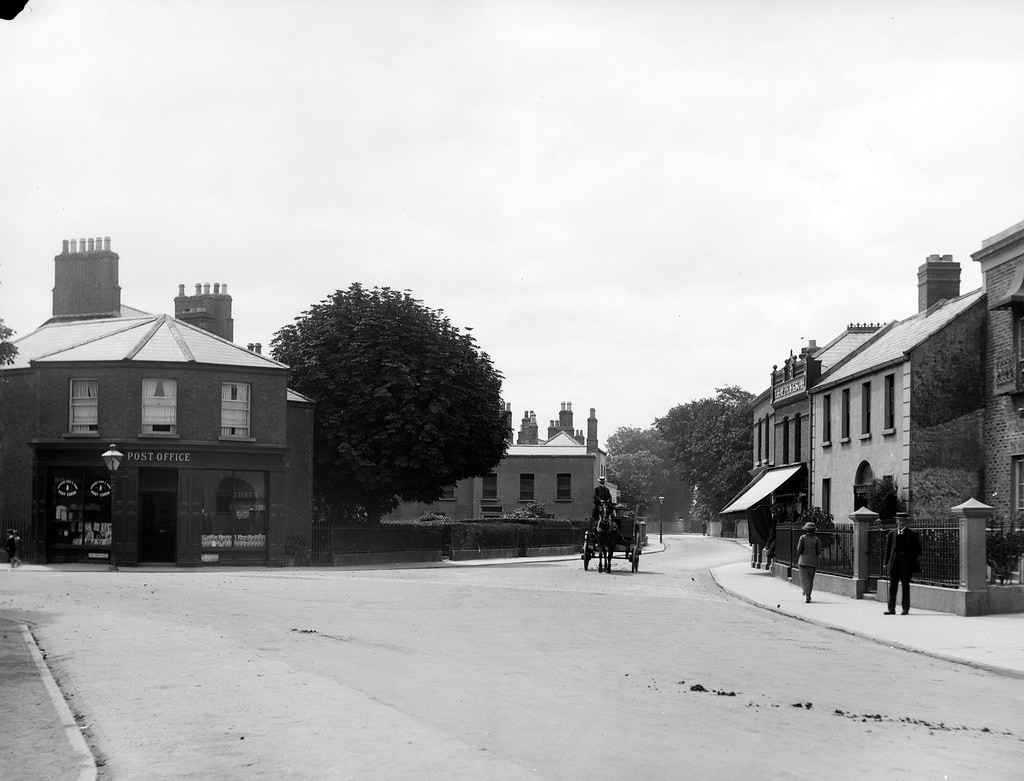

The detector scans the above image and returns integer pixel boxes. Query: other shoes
[901,610,909,615]
[883,610,895,614]
[805,594,811,603]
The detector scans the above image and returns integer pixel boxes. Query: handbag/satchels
[799,542,804,553]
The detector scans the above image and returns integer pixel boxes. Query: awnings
[718,465,806,514]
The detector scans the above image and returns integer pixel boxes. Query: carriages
[582,495,642,575]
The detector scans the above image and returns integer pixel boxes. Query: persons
[702,521,707,535]
[592,477,614,529]
[884,513,919,616]
[5,529,21,568]
[797,522,822,603]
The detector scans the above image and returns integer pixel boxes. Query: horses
[594,495,619,572]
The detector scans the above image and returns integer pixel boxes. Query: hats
[802,522,818,532]
[598,477,607,482]
[894,512,911,519]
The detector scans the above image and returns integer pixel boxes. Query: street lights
[658,490,664,544]
[100,443,124,572]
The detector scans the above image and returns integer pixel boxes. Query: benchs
[589,516,634,540]
